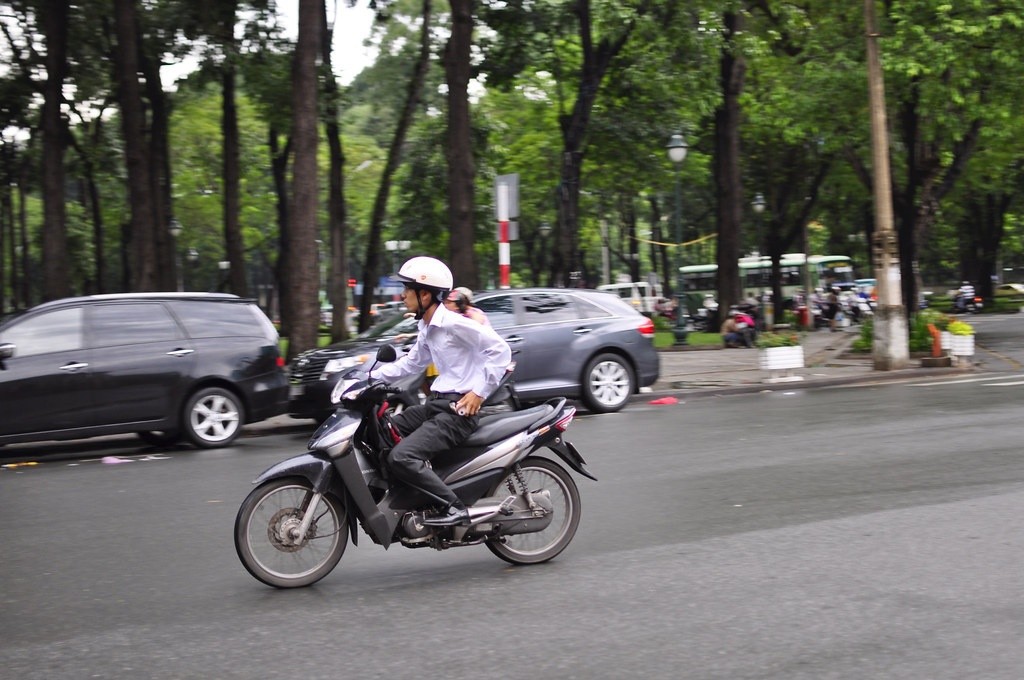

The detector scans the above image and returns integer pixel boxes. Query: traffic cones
[931,330,943,358]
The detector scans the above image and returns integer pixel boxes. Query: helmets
[454,287,473,301]
[445,291,468,304]
[389,256,453,292]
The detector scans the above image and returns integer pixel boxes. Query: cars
[288,287,662,428]
[0,292,290,451]
[516,288,655,346]
[595,282,662,318]
[998,283,1024,291]
[854,279,877,290]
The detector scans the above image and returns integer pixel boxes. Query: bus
[678,253,855,322]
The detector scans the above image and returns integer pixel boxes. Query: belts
[431,391,466,402]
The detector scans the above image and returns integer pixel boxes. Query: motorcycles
[384,334,525,419]
[233,344,598,590]
[952,295,983,314]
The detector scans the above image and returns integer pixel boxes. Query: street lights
[666,129,695,346]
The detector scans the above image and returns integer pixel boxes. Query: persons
[364,256,511,526]
[654,298,685,329]
[847,286,878,322]
[954,281,975,309]
[721,310,758,348]
[817,271,843,332]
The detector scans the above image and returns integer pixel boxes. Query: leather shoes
[420,505,471,527]
